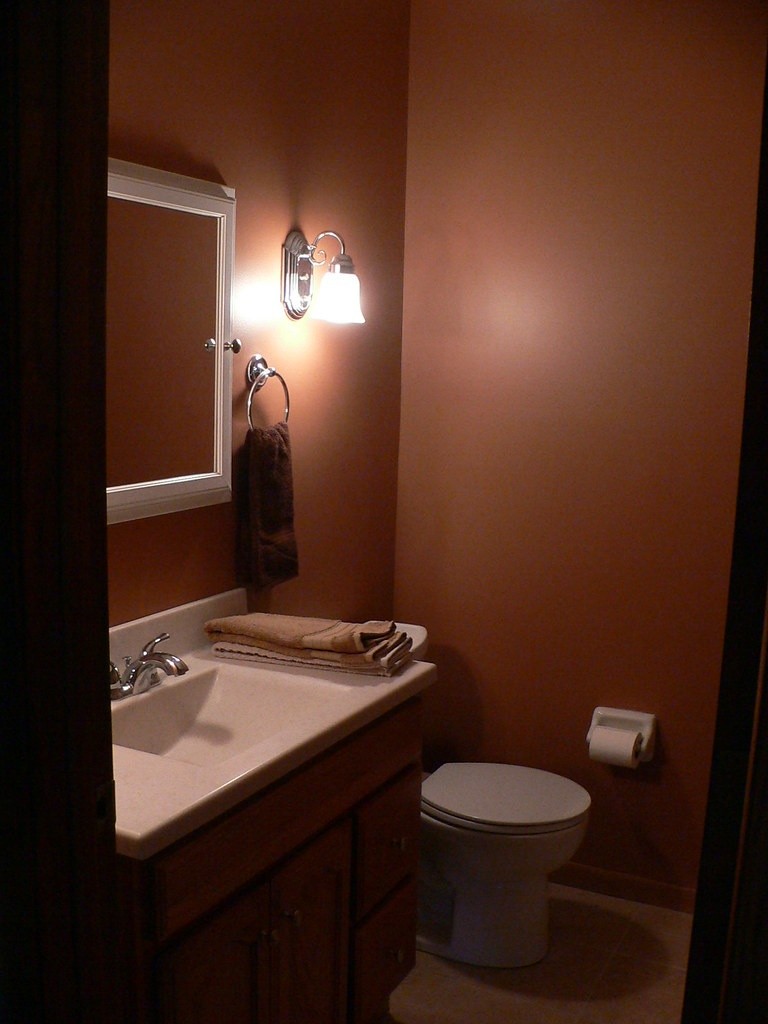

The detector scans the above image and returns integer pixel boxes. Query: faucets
[121,651,189,694]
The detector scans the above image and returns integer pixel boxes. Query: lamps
[280,230,365,324]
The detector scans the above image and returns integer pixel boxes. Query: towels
[202,611,415,679]
[233,422,300,593]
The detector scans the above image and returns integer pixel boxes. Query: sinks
[112,664,352,769]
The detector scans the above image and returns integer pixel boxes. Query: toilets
[360,619,591,969]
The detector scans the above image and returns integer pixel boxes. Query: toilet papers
[589,725,644,769]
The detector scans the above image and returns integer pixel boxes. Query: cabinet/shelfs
[120,696,427,1024]
